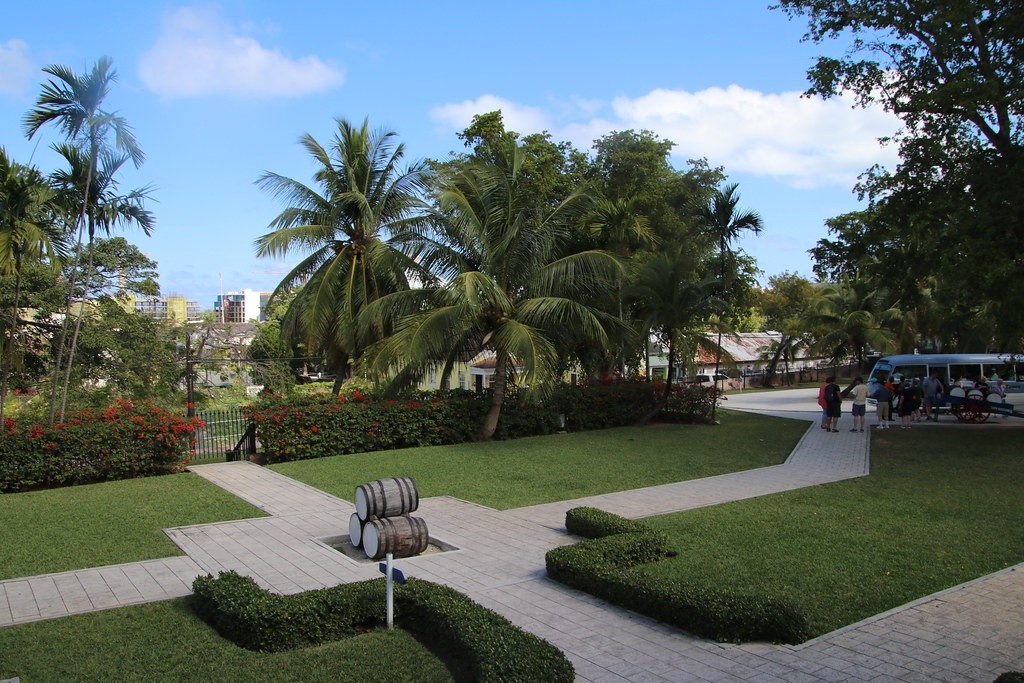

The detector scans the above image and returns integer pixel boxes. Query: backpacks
[824,386,835,403]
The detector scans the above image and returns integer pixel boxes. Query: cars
[697,374,730,384]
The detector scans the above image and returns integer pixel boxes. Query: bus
[865,353,1024,412]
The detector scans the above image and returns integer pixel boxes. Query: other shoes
[820,425,826,429]
[832,429,840,432]
[826,428,831,432]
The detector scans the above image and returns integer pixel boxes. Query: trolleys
[942,384,1024,424]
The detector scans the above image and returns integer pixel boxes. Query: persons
[819,378,830,429]
[849,377,870,432]
[872,380,891,429]
[887,376,897,420]
[825,375,842,432]
[897,371,1005,430]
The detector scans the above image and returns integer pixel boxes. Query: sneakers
[883,426,889,429]
[900,425,906,428]
[859,428,864,432]
[849,428,858,432]
[908,426,911,428]
[876,426,884,429]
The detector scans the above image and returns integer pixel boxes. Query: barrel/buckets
[355,477,419,522]
[362,516,428,559]
[349,513,370,548]
